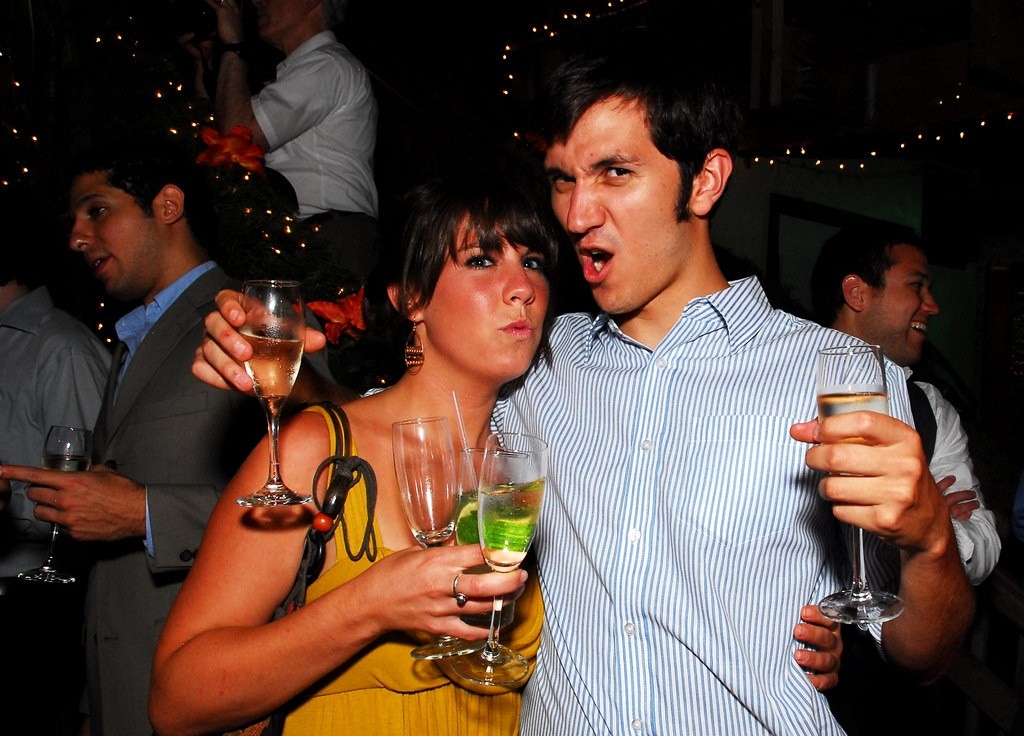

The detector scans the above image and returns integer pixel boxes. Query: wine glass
[235,278,313,511]
[818,344,905,627]
[16,424,93,587]
[451,433,549,687]
[392,416,484,665]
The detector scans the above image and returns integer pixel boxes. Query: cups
[455,448,528,629]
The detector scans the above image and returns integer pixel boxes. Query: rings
[453,575,471,606]
[218,0,225,3]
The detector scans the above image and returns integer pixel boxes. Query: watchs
[222,42,250,60]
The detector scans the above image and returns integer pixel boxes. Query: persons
[149,167,845,736]
[189,37,973,736]
[792,223,1024,736]
[0,1,378,736]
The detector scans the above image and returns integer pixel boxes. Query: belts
[299,209,364,226]
